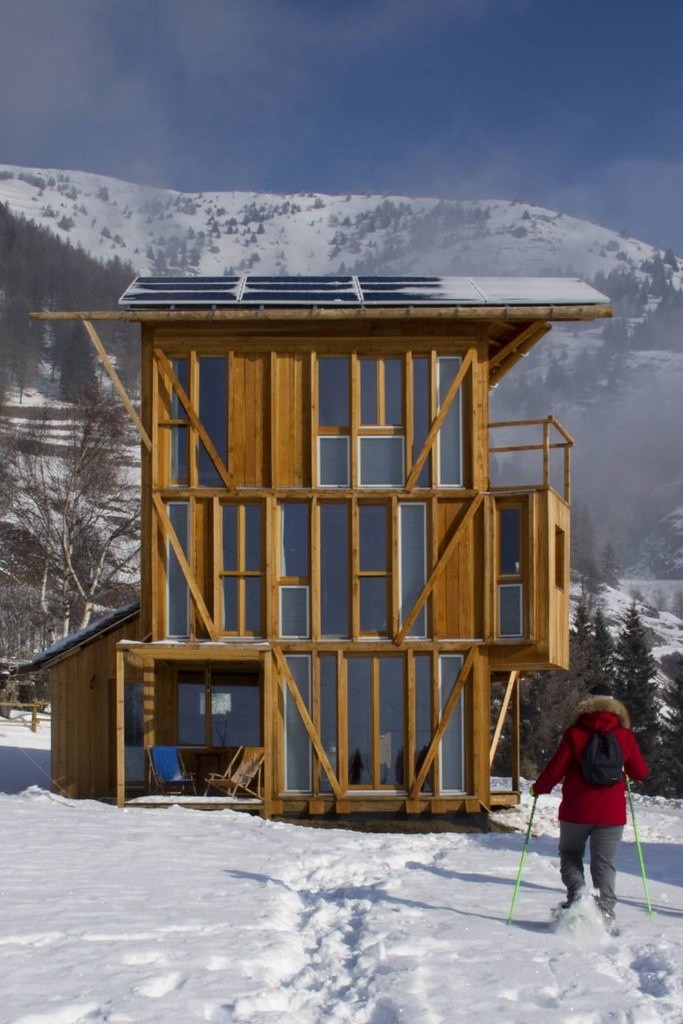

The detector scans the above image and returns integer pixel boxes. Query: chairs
[144,744,197,796]
[203,745,266,801]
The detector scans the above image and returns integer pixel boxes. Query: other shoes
[605,925,619,937]
[561,893,584,909]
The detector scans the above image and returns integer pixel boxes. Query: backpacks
[571,722,624,786]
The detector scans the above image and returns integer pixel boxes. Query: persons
[529,683,649,926]
